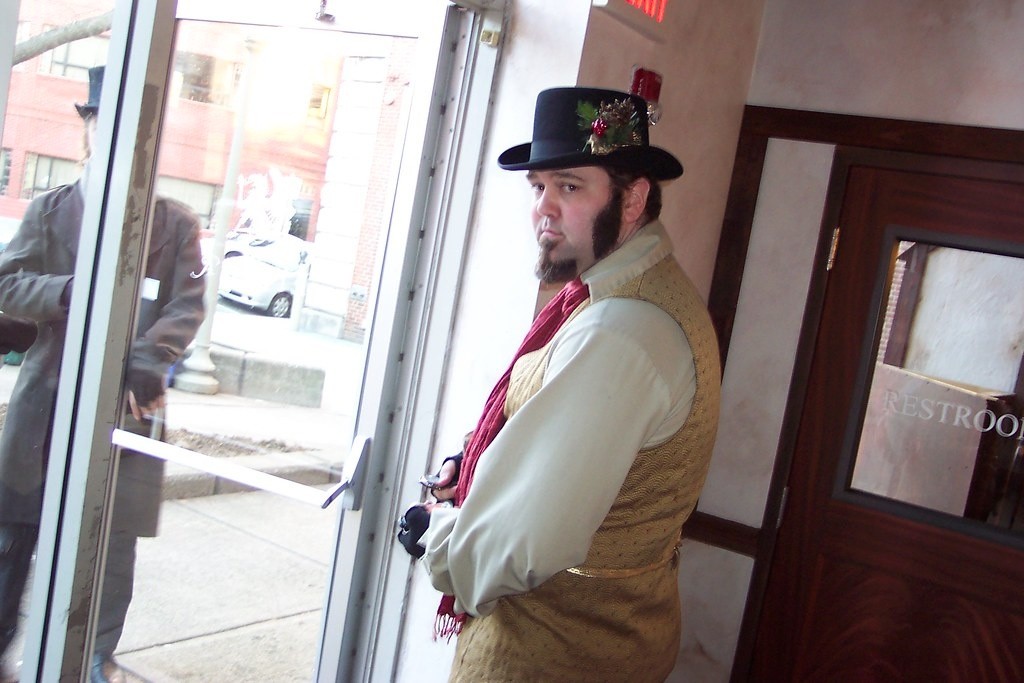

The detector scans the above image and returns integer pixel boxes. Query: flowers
[573,92,644,157]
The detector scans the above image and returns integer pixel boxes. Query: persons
[398,86,721,683]
[0,64,207,683]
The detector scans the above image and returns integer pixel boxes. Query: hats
[74,66,105,118]
[497,86,684,181]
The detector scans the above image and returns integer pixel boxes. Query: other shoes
[91,653,126,683]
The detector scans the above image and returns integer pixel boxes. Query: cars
[191,208,313,320]
